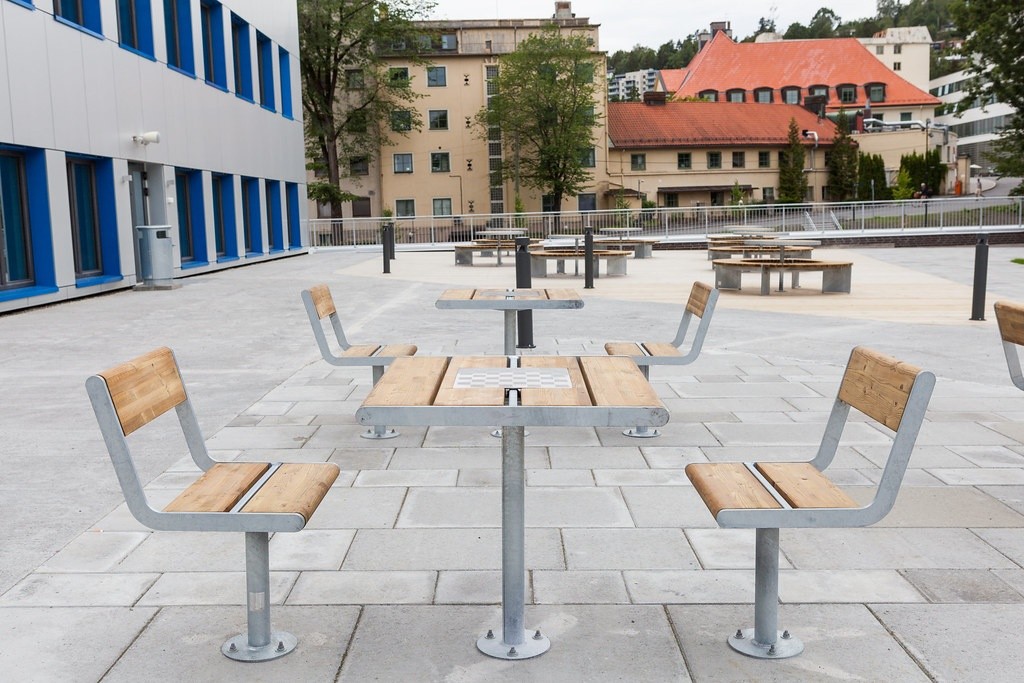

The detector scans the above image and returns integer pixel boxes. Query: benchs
[458,235,657,279]
[708,236,853,297]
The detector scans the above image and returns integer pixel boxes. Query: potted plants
[672,212,684,223]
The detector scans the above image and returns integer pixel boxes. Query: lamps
[468,200,475,212]
[465,115,471,127]
[466,158,473,171]
[132,131,160,145]
[464,73,469,85]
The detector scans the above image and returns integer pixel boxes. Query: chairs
[606,280,718,437]
[684,345,937,660]
[303,285,419,438]
[995,304,1024,392]
[86,345,340,659]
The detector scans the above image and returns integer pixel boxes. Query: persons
[917,183,928,207]
[976,179,985,201]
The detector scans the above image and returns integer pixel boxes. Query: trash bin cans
[136,225,173,285]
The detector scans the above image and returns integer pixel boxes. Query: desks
[357,354,671,660]
[485,228,528,256]
[435,287,583,356]
[551,234,609,275]
[724,225,822,290]
[601,227,642,251]
[475,231,526,263]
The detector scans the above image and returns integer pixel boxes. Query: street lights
[926,118,931,158]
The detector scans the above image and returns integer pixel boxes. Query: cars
[979,168,1003,176]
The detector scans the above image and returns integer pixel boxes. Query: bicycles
[912,193,933,207]
